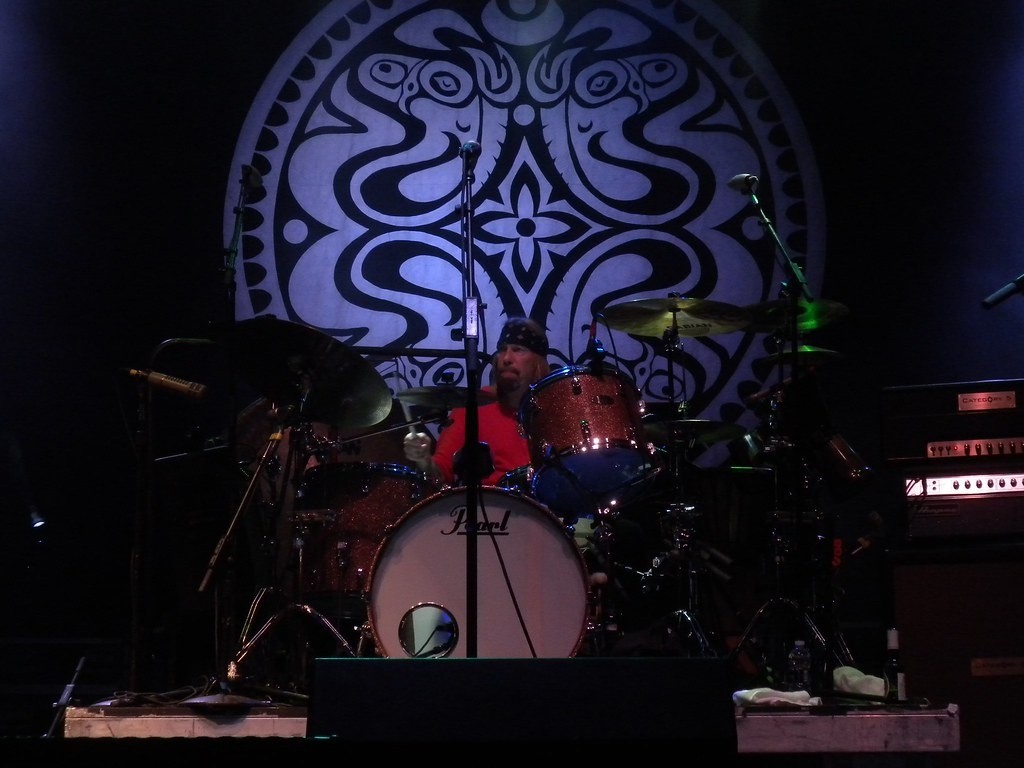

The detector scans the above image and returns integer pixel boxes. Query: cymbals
[395,384,497,409]
[740,293,854,337]
[216,316,395,429]
[769,344,840,362]
[595,296,756,340]
[640,417,747,449]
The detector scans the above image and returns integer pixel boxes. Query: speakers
[879,543,1024,766]
[305,657,739,768]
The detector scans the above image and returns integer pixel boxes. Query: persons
[404,317,553,486]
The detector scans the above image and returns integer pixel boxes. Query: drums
[513,365,656,506]
[365,483,592,659]
[294,460,452,622]
[495,462,540,500]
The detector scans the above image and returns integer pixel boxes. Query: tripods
[111,365,361,708]
[658,430,860,672]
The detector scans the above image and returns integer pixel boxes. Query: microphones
[265,405,296,420]
[585,314,597,360]
[749,368,816,402]
[459,141,482,159]
[124,366,208,402]
[241,164,264,190]
[725,174,759,192]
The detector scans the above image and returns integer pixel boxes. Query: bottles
[881,623,908,707]
[789,641,811,694]
